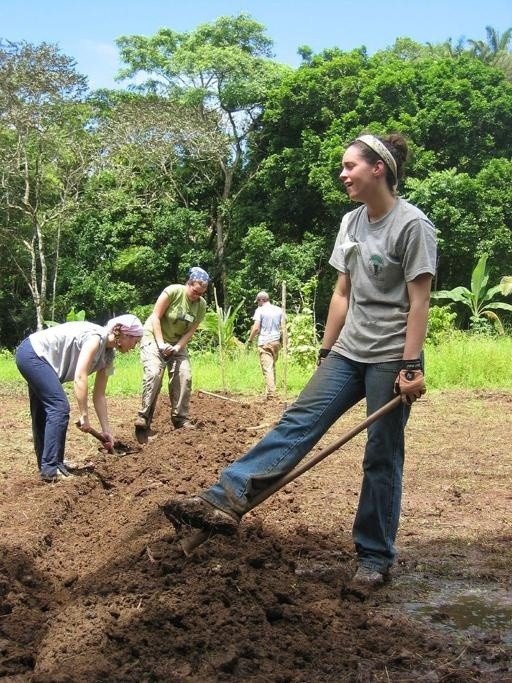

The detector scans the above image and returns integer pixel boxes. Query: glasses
[190,285,205,296]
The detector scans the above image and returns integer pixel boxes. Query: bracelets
[247,340,252,343]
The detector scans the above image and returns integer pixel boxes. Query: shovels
[135,348,174,444]
[75,420,140,456]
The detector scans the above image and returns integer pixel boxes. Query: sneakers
[135,417,148,429]
[41,459,80,483]
[166,496,238,537]
[177,421,196,430]
[352,566,385,589]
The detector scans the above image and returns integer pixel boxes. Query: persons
[245,291,283,400]
[15,314,143,481]
[162,134,437,583]
[134,266,209,429]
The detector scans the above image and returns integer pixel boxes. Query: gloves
[245,340,252,351]
[393,359,425,406]
